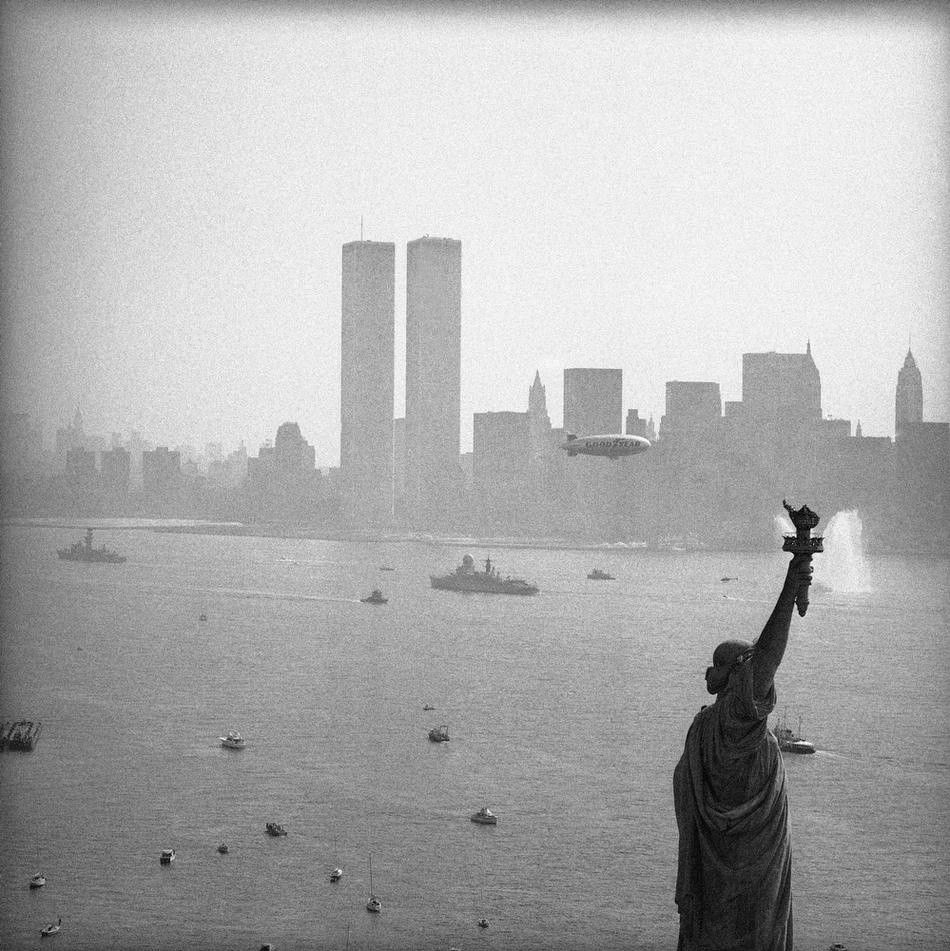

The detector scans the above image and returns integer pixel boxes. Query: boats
[219,729,247,749]
[771,707,817,753]
[57,527,128,563]
[423,702,452,741]
[40,918,61,935]
[160,849,177,864]
[362,590,388,605]
[430,552,539,596]
[218,842,229,853]
[472,807,497,825]
[587,568,616,580]
[380,565,395,570]
[266,822,289,836]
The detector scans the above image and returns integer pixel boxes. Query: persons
[673,552,815,951]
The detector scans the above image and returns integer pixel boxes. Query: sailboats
[478,889,490,927]
[330,834,342,881]
[29,829,46,887]
[364,851,381,911]
